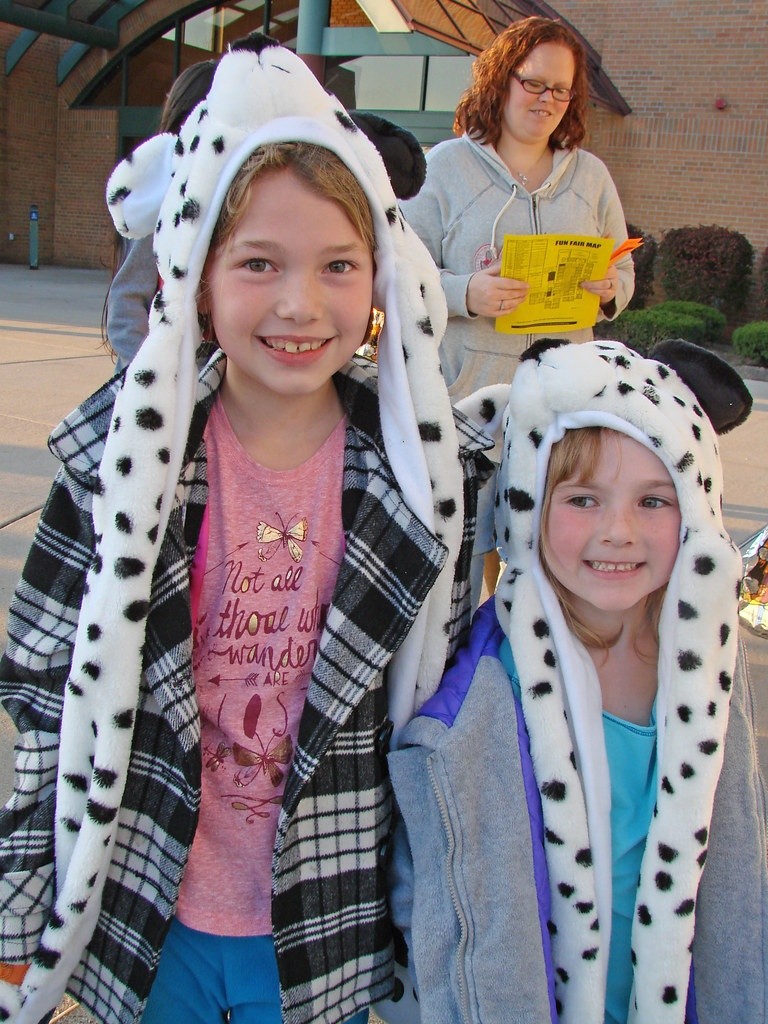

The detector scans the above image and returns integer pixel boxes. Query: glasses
[511,68,580,102]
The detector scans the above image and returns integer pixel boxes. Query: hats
[445,335,754,1024]
[4,33,463,1024]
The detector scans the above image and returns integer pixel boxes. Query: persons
[398,16,635,602]
[385,337,767,1024]
[0,34,464,1023]
